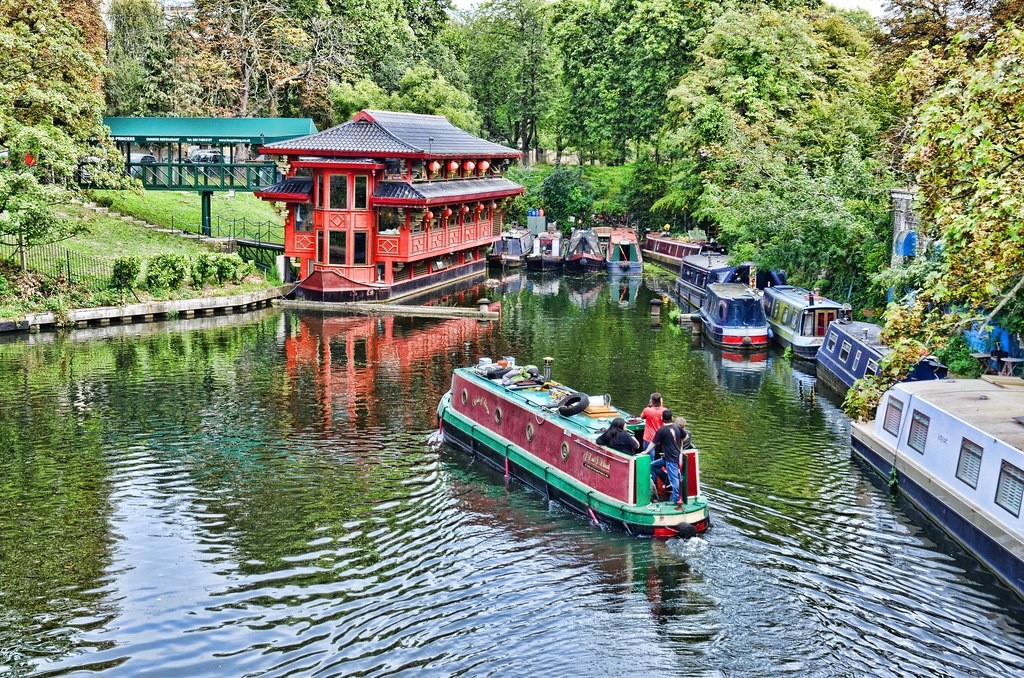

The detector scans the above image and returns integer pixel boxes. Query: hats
[662,410,671,420]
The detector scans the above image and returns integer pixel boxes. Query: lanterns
[464,161,475,173]
[442,208,452,220]
[476,204,484,212]
[423,210,433,221]
[447,161,459,172]
[460,205,469,215]
[478,161,490,172]
[429,162,439,173]
[490,203,496,210]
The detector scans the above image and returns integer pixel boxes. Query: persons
[675,417,692,451]
[642,410,689,504]
[641,392,671,491]
[596,418,640,455]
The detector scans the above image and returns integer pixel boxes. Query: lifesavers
[557,393,590,417]
[487,367,513,379]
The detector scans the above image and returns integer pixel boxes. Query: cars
[66,147,230,186]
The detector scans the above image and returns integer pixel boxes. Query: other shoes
[665,502,678,506]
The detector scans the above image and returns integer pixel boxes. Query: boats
[640,223,729,268]
[674,250,787,311]
[436,354,711,538]
[699,282,771,351]
[815,305,950,416]
[845,371,1024,603]
[760,283,853,359]
[488,222,645,274]
